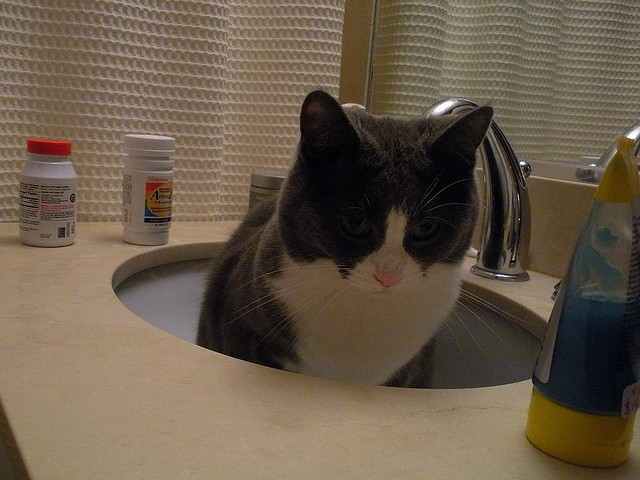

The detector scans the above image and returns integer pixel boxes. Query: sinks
[111,242,548,389]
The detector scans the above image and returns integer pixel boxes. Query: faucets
[419,98,532,282]
[551,137,640,303]
[577,122,640,182]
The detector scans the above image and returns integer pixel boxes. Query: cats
[195,90,494,387]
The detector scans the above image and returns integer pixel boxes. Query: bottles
[120,133,176,245]
[248,171,286,209]
[18,138,79,247]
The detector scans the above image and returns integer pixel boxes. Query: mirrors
[365,0,640,185]
